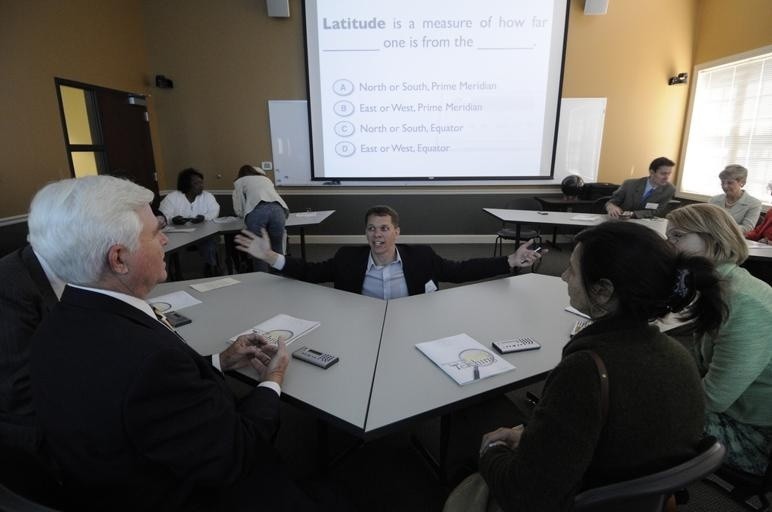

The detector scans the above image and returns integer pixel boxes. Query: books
[227,314,321,354]
[145,289,203,314]
[414,333,516,386]
[160,226,195,233]
[569,319,592,336]
[744,238,771,250]
[566,306,592,321]
[211,215,237,224]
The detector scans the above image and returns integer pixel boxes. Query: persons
[604,158,676,223]
[660,203,771,476]
[234,205,549,300]
[439,220,731,511]
[158,168,221,275]
[231,165,290,275]
[2,178,77,501]
[27,174,341,512]
[742,182,771,248]
[112,169,166,230]
[705,164,764,235]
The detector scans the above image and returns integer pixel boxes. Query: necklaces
[724,200,737,207]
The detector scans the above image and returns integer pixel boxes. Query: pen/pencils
[520,247,542,264]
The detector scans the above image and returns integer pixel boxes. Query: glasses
[668,231,696,245]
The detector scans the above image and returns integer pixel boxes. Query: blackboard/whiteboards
[267,97,607,187]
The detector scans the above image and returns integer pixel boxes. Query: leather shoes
[676,488,689,505]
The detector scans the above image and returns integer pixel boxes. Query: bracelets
[480,441,510,457]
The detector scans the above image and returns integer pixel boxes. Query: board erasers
[323,181,340,186]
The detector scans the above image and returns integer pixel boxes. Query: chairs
[567,433,724,510]
[494,197,541,257]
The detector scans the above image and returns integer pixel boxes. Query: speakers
[267,0,290,17]
[584,0,608,15]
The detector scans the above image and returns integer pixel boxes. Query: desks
[218,207,336,269]
[534,196,681,253]
[483,206,772,280]
[141,269,387,508]
[159,220,219,279]
[365,270,686,510]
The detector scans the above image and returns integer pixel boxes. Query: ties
[150,304,185,341]
[640,188,655,203]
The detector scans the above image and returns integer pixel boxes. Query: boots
[204,262,223,277]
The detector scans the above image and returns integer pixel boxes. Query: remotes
[521,247,541,263]
